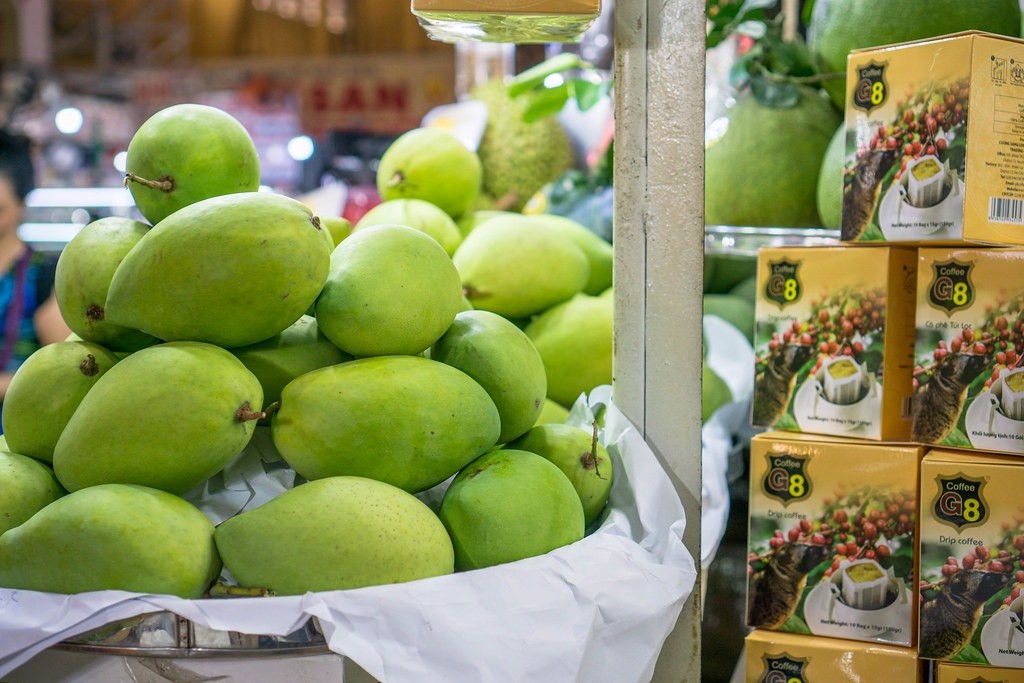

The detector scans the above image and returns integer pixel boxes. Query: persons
[0,128,73,436]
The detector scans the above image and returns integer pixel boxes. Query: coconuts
[707,0,1024,232]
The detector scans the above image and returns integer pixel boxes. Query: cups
[1004,593,1024,654]
[824,568,902,637]
[894,169,955,235]
[985,376,1024,444]
[809,367,873,431]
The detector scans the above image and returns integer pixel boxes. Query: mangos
[1,103,759,602]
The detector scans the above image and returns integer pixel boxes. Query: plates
[878,178,964,239]
[965,391,1024,453]
[981,607,1024,668]
[804,580,912,646]
[793,377,882,441]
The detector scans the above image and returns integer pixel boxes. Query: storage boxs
[749,245,913,443]
[745,438,924,648]
[937,662,1024,683]
[744,629,919,683]
[917,451,1024,668]
[912,247,1024,456]
[840,30,1023,249]
